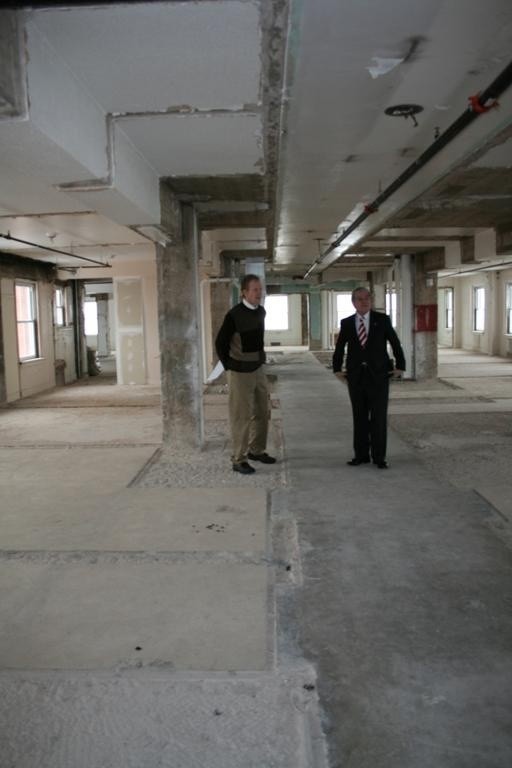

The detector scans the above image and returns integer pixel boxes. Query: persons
[333,288,406,469]
[214,275,276,474]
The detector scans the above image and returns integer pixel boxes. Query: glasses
[355,297,369,301]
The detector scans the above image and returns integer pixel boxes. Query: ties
[357,317,368,349]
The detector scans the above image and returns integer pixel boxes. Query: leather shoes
[373,458,389,469]
[346,456,370,466]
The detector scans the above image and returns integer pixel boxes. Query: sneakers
[233,462,256,474]
[246,452,277,464]
